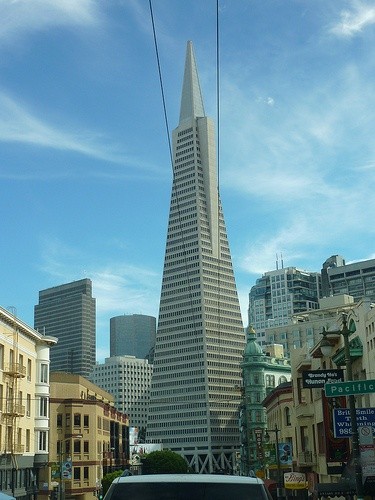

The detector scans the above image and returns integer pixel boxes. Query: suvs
[101,474,269,500]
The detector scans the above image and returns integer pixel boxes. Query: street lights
[264,425,282,500]
[59,435,83,500]
[318,314,365,500]
